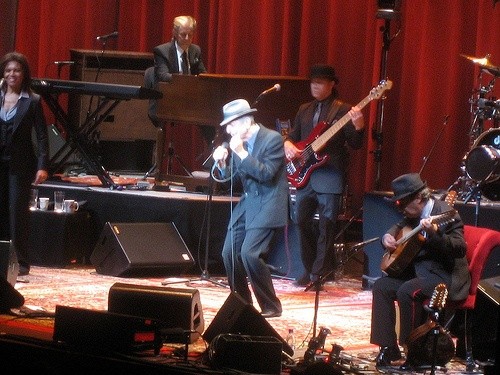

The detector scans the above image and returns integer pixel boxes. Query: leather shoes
[401,359,411,370]
[375,344,401,365]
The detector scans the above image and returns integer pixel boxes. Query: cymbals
[457,52,500,76]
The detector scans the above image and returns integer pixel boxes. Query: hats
[391,173,425,203]
[309,65,339,81]
[220,98,258,127]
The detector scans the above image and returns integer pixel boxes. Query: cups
[64,200,79,213]
[54,191,65,212]
[39,198,49,210]
[29,188,40,210]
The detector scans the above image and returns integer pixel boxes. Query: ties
[313,103,323,128]
[180,51,188,74]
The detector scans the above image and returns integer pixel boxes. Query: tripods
[161,94,268,289]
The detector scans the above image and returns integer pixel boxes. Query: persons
[147,16,216,191]
[284,64,365,286]
[213,99,288,317]
[371,173,474,371]
[0,52,50,277]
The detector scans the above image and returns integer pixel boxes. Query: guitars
[405,282,456,372]
[285,77,393,191]
[380,209,459,277]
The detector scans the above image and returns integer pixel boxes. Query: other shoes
[260,310,281,317]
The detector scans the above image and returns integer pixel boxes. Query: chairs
[412,224,500,359]
[141,66,194,181]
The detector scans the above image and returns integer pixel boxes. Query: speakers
[0,240,25,312]
[89,223,196,278]
[67,49,159,142]
[470,276,500,362]
[54,282,294,375]
[48,124,82,164]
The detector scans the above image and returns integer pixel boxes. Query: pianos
[154,72,316,198]
[29,77,165,191]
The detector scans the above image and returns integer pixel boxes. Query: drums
[463,145,500,184]
[469,127,500,202]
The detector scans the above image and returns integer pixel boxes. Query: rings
[43,178,45,181]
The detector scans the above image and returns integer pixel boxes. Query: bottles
[285,328,296,356]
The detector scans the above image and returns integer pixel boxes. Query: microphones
[53,61,74,65]
[0,77,5,88]
[96,32,119,40]
[261,83,281,95]
[356,237,380,248]
[211,142,229,172]
[428,283,449,334]
